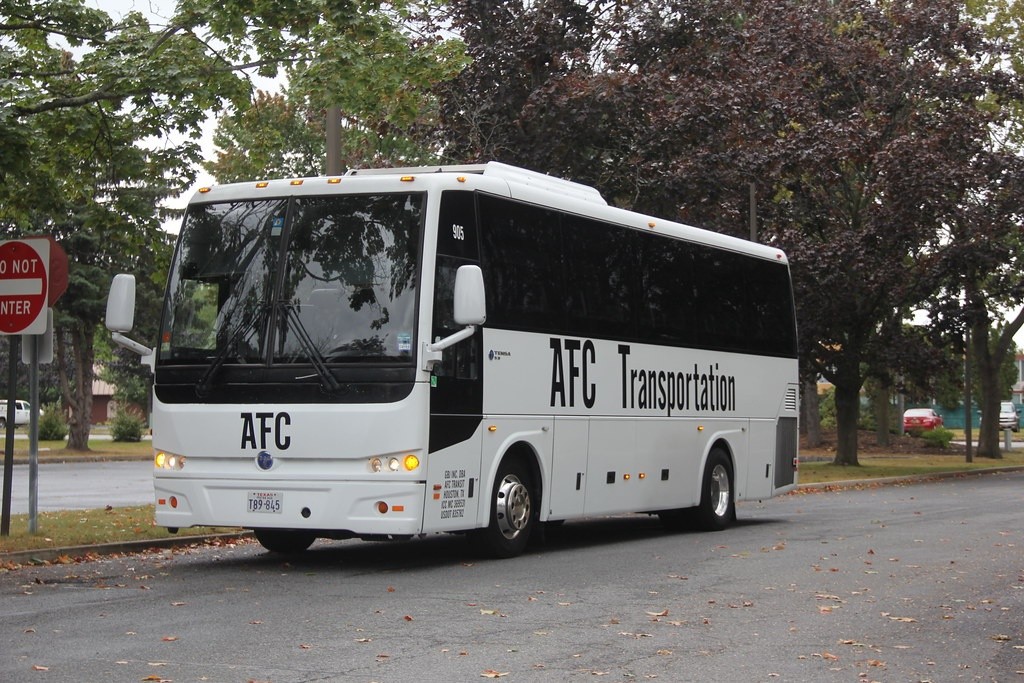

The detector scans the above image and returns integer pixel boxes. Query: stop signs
[0,237,53,337]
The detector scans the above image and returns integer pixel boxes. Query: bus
[104,159,801,559]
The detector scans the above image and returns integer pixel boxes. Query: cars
[977,401,1022,433]
[902,409,945,432]
